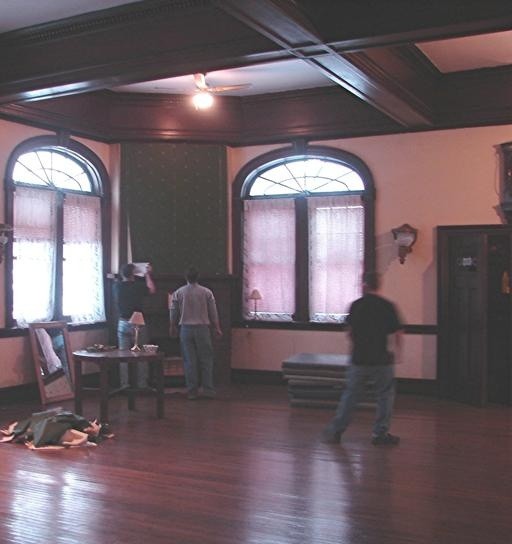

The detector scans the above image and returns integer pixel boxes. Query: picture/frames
[27,321,79,405]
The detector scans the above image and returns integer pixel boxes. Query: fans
[154,77,258,97]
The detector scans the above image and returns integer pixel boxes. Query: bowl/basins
[142,345,158,352]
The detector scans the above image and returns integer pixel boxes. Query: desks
[73,347,172,425]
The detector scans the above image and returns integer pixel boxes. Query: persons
[319,268,404,447]
[112,262,159,389]
[167,265,226,401]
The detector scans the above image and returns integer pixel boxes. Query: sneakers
[186,387,217,400]
[371,431,401,446]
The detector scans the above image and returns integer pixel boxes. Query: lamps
[129,309,146,350]
[251,287,261,322]
[191,92,214,111]
[391,219,418,263]
